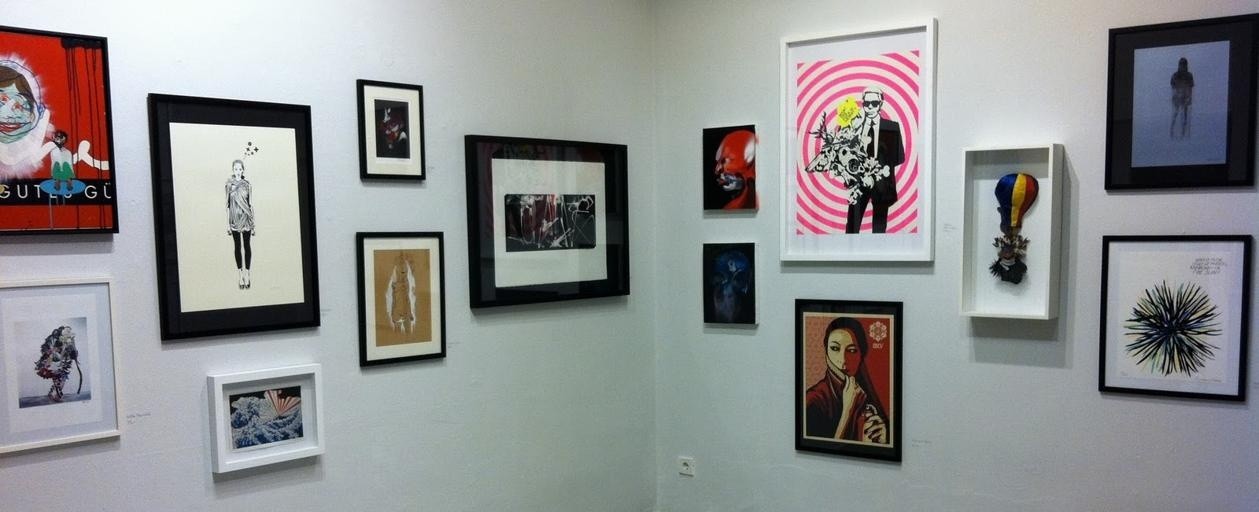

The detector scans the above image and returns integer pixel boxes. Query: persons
[839,85,906,234]
[1165,55,1194,146]
[805,317,889,444]
[224,159,256,290]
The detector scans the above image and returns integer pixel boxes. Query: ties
[867,120,874,157]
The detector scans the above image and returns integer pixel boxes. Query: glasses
[862,101,880,107]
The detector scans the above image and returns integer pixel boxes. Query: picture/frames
[958,143,1064,321]
[1105,13,1259,190]
[206,363,325,474]
[0,25,120,234]
[702,125,756,211]
[779,17,937,263]
[0,277,121,455]
[463,134,629,308]
[147,93,321,342]
[1098,234,1252,401]
[356,232,446,366]
[794,299,903,462]
[702,242,756,326]
[356,79,426,180]
